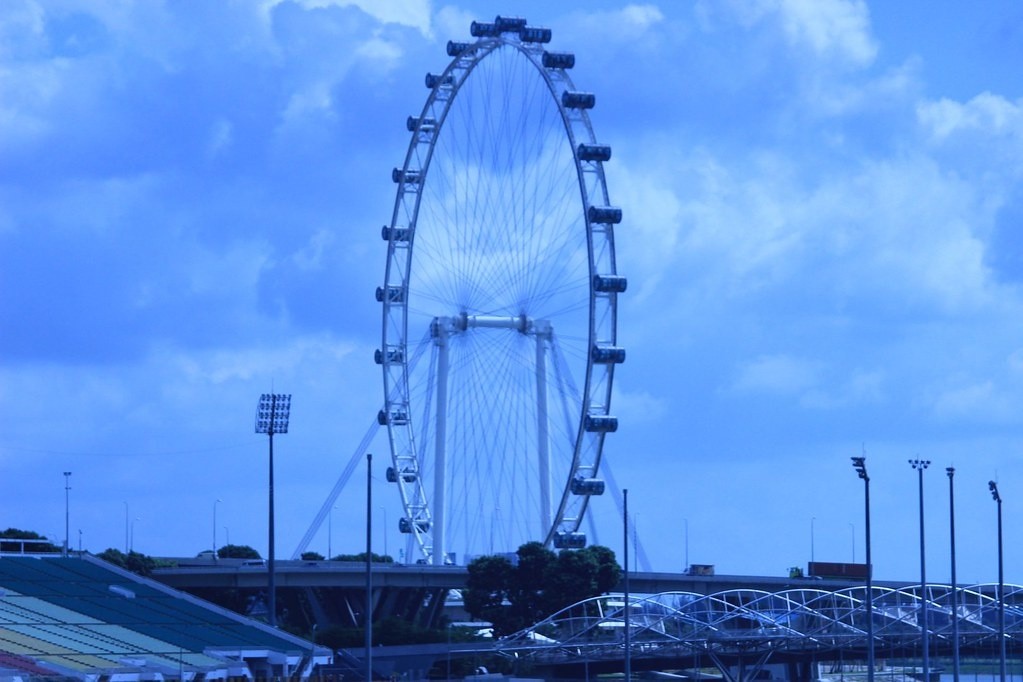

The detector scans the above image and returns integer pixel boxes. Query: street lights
[682,516,691,572]
[631,511,641,573]
[212,498,223,558]
[379,505,387,564]
[64,470,73,556]
[909,459,930,682]
[131,518,141,551]
[222,526,230,558]
[850,456,875,682]
[254,392,293,627]
[945,463,962,682]
[79,529,83,556]
[329,504,340,561]
[809,517,817,579]
[849,524,857,565]
[122,501,128,556]
[988,480,1006,682]
